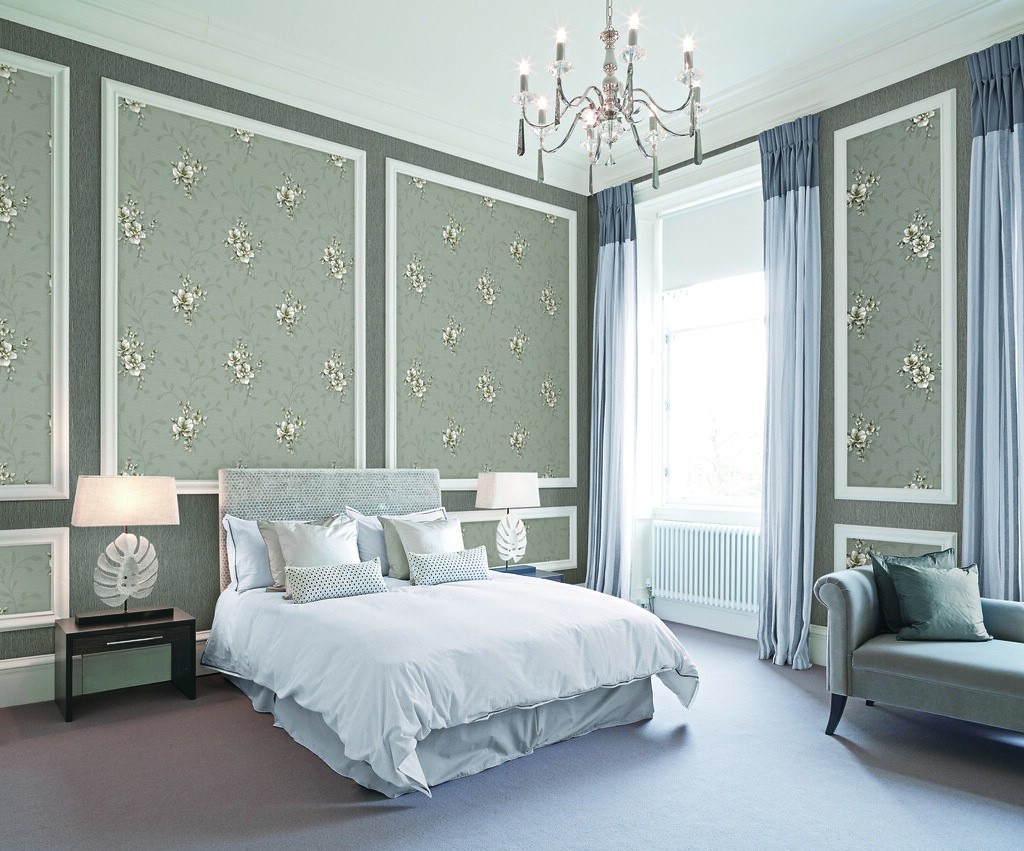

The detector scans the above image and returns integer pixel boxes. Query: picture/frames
[450,506,577,571]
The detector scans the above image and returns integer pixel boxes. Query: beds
[200,468,699,798]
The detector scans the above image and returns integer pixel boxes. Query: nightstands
[533,569,565,583]
[54,607,197,723]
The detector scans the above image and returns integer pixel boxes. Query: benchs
[814,564,1024,737]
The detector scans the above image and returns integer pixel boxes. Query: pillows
[345,505,447,576]
[885,562,993,642]
[257,515,340,592]
[283,556,387,605]
[391,519,465,554]
[377,506,447,579]
[406,544,493,584]
[867,547,956,633]
[274,518,361,601]
[222,514,275,592]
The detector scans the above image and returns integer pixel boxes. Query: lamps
[475,470,541,576]
[516,0,703,197]
[70,476,181,626]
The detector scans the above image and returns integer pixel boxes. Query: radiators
[649,518,760,614]
